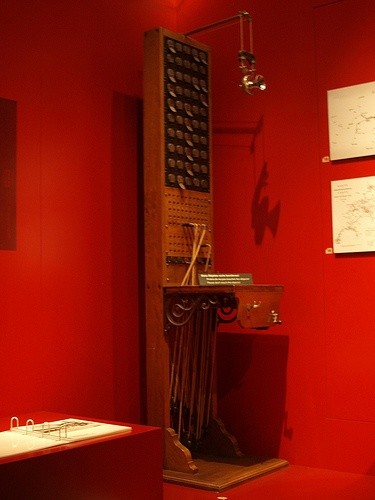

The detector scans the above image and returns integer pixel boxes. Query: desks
[0,414,163,500]
